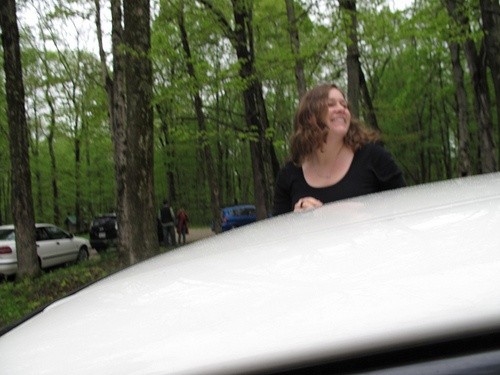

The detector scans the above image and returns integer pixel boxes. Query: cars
[211,205,272,234]
[0,170,500,375]
[0,221,91,281]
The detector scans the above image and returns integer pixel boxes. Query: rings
[300,201,304,208]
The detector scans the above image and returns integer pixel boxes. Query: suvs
[87,212,120,256]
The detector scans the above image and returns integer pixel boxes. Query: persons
[176,202,189,246]
[157,200,178,248]
[273,83,406,218]
